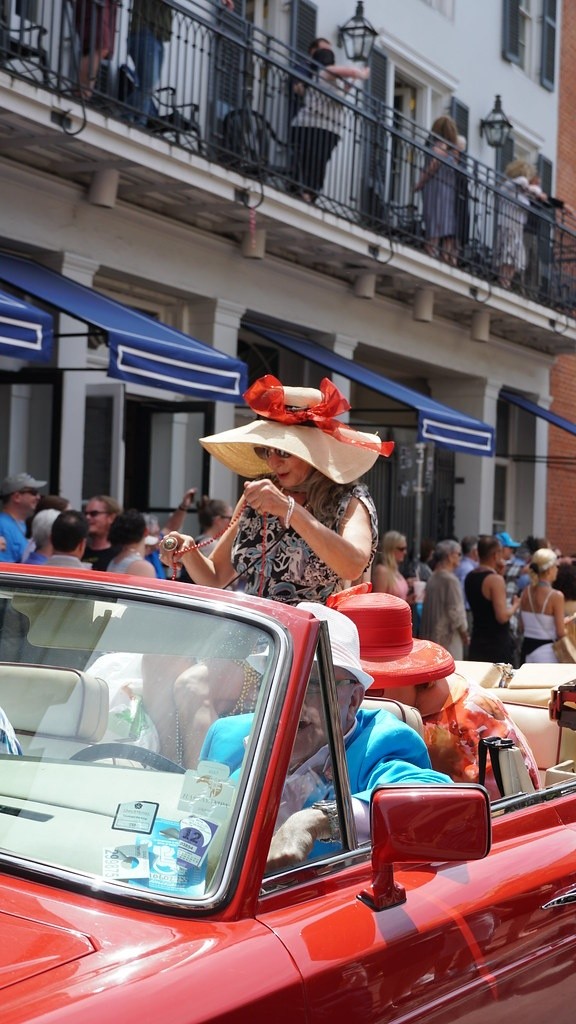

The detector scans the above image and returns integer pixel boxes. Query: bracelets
[285,495,295,529]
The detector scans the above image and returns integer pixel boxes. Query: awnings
[500,388,576,465]
[0,290,56,363]
[240,319,496,459]
[0,251,248,404]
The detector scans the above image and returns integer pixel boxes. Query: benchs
[85,650,575,787]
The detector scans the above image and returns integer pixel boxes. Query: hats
[0,473,47,495]
[326,582,455,690]
[497,533,522,548]
[198,373,394,485]
[530,548,556,569]
[245,600,373,695]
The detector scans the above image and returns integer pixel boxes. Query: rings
[161,537,178,552]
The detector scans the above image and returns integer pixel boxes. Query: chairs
[365,173,425,252]
[118,66,202,154]
[460,238,576,316]
[0,0,50,87]
[224,109,299,193]
[0,660,116,765]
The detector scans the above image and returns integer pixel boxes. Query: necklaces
[172,499,267,600]
[278,486,313,509]
[176,655,259,769]
[122,545,141,557]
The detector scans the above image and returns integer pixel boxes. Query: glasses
[84,510,112,517]
[19,489,39,496]
[394,546,406,552]
[252,444,292,461]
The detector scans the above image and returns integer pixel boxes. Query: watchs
[311,800,340,844]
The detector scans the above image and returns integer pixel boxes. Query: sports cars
[0,562,575,1024]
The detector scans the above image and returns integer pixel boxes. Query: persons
[491,159,576,291]
[142,374,378,771]
[412,116,459,262]
[66,0,173,128]
[197,593,541,892]
[285,37,371,202]
[0,472,575,672]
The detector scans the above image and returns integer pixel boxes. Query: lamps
[336,0,379,63]
[481,94,513,149]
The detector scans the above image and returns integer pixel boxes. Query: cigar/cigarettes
[298,719,308,732]
